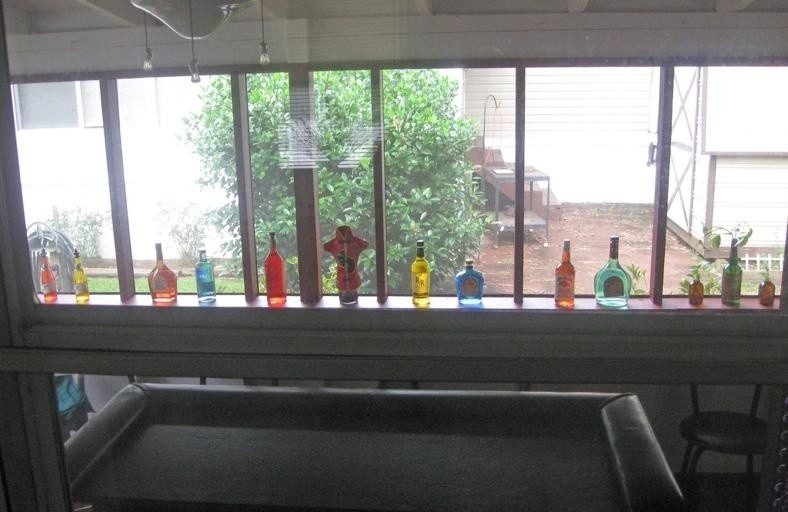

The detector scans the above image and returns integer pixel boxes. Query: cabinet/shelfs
[485,165,551,248]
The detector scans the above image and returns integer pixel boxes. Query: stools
[679,410,768,511]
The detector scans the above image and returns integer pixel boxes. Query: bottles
[263,231,288,308]
[593,235,632,308]
[719,238,743,306]
[411,238,430,305]
[453,259,485,305]
[554,238,575,311]
[194,250,216,304]
[38,247,58,304]
[70,248,91,304]
[688,278,705,306]
[147,244,178,303]
[757,279,776,306]
